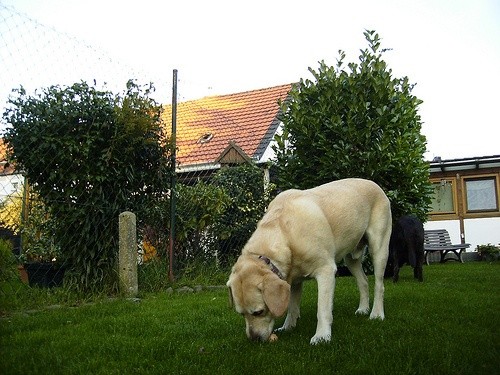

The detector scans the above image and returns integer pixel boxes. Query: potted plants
[474,244,500,261]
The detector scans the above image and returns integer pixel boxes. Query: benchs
[423,228,470,266]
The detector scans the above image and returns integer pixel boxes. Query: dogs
[384,216,424,283]
[226,178,392,346]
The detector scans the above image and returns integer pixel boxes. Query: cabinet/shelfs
[18,263,63,288]
